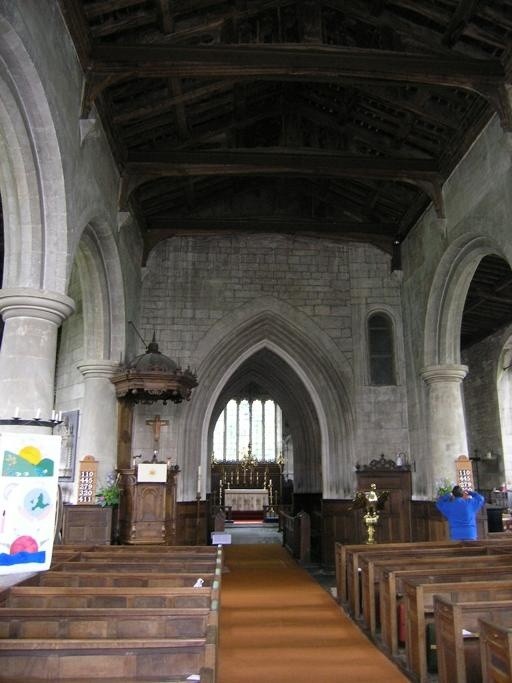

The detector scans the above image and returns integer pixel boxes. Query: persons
[435,485,485,541]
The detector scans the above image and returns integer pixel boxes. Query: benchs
[0,541,224,683]
[335,538,512,683]
